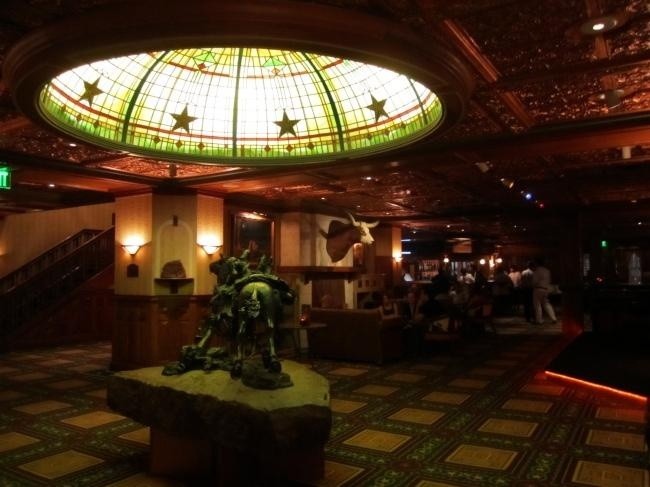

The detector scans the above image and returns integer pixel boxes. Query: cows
[326,212,380,263]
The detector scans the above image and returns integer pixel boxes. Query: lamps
[120,243,141,255]
[199,244,222,254]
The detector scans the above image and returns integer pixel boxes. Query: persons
[381,256,555,325]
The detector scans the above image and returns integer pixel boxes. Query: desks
[278,322,329,367]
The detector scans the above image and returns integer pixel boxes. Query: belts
[534,286,548,290]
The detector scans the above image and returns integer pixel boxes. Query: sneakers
[528,318,558,329]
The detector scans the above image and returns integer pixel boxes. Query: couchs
[304,305,407,361]
